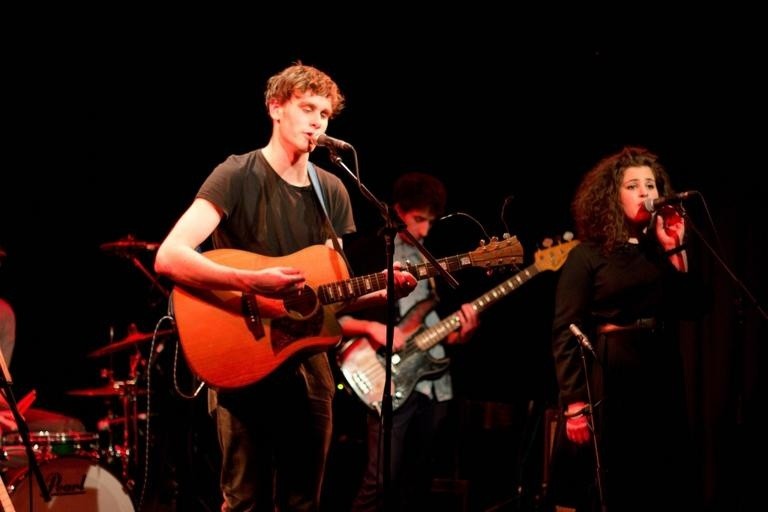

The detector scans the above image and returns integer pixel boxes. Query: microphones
[312,133,353,152]
[645,190,698,212]
[569,324,598,356]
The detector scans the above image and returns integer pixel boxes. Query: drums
[0,457,137,512]
[2,429,102,461]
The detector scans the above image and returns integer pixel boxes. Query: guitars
[168,233,524,390]
[336,233,581,412]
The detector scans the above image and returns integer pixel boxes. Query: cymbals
[69,387,148,396]
[91,329,173,357]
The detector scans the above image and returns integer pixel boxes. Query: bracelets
[562,405,589,417]
[663,242,688,256]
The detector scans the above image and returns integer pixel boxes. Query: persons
[552,146,707,511]
[335,174,479,511]
[153,62,418,512]
